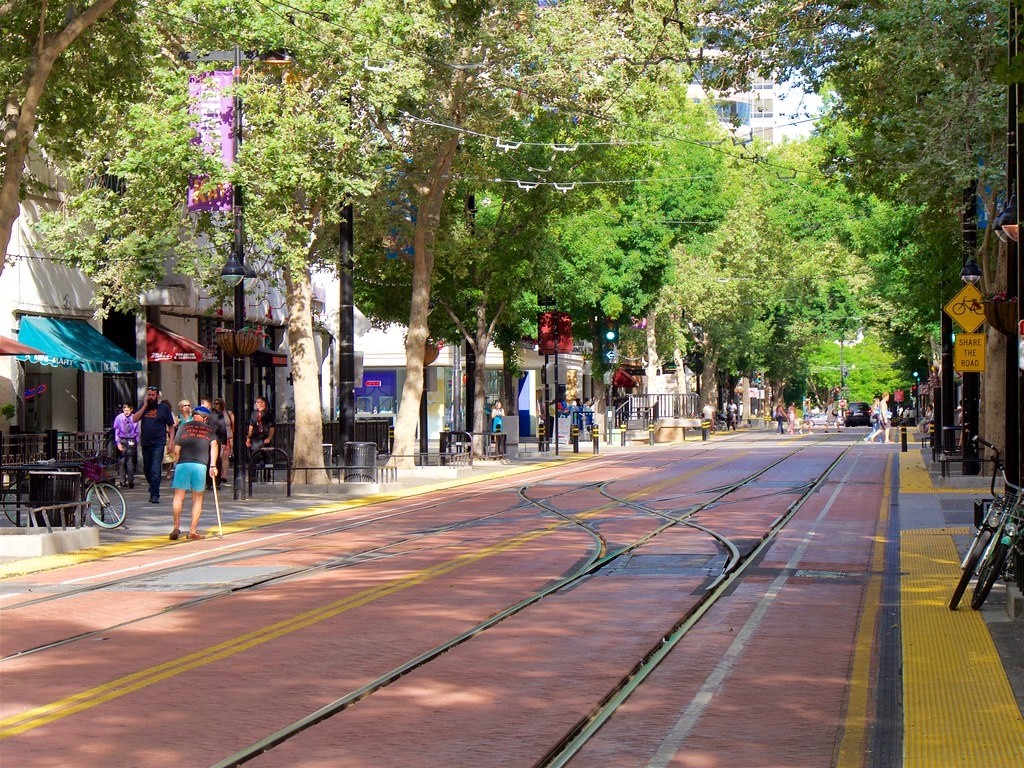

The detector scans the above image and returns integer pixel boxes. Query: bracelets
[210,466,216,468]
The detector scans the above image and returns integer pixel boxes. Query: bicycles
[949,436,1024,612]
[2,436,128,529]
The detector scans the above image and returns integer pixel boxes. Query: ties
[258,411,263,433]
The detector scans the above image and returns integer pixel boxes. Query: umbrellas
[0,336,45,355]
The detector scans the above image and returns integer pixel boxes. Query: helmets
[191,406,210,419]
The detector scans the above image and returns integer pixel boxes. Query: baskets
[995,490,1024,521]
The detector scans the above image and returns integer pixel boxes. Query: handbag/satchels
[870,414,877,423]
[881,420,891,430]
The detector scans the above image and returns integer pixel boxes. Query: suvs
[844,403,872,427]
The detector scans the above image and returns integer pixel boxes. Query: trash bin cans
[343,442,378,482]
[30,470,80,527]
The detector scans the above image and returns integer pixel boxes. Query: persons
[864,391,891,444]
[491,401,505,442]
[169,406,220,540]
[825,398,841,432]
[954,400,964,451]
[897,403,910,418]
[775,400,813,435]
[132,386,174,503]
[918,403,933,434]
[113,402,139,489]
[549,398,595,441]
[702,401,716,432]
[723,398,737,431]
[160,397,234,490]
[246,396,276,482]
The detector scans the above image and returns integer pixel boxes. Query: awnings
[17,316,142,373]
[252,348,288,367]
[145,321,218,362]
[612,369,639,387]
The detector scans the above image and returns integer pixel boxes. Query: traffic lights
[606,317,617,341]
[834,386,840,399]
[911,386,917,397]
[917,377,921,382]
[756,371,764,386]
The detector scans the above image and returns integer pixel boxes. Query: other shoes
[864,437,894,444]
[838,430,843,432]
[129,483,134,488]
[169,529,180,540]
[148,495,160,502]
[208,484,221,490]
[809,432,814,434]
[186,532,205,539]
[799,430,803,434]
[221,478,227,482]
[251,477,258,481]
[825,430,829,433]
[120,482,127,487]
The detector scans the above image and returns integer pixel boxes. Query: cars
[809,412,845,426]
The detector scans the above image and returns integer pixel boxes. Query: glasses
[184,404,190,407]
[148,386,159,391]
[214,403,220,405]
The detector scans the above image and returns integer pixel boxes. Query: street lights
[177,45,294,501]
